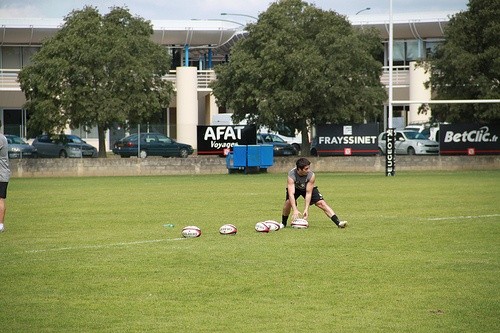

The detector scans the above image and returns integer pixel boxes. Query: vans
[404,122,439,142]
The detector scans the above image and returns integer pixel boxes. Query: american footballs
[220,224,237,235]
[292,219,310,228]
[255,223,269,231]
[183,226,202,238]
[265,221,278,231]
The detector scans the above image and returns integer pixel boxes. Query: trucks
[440,124,500,155]
[309,123,379,156]
[197,124,258,158]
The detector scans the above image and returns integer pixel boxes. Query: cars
[6,135,38,159]
[378,129,440,155]
[113,132,194,159]
[256,134,299,156]
[32,133,97,158]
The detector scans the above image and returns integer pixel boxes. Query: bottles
[163,224,174,228]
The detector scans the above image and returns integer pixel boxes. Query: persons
[0,119,12,232]
[280,158,347,229]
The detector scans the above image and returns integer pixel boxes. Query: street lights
[355,7,371,15]
[220,12,258,21]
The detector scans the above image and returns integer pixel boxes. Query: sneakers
[338,221,348,228]
[280,223,285,229]
[0,224,4,232]
